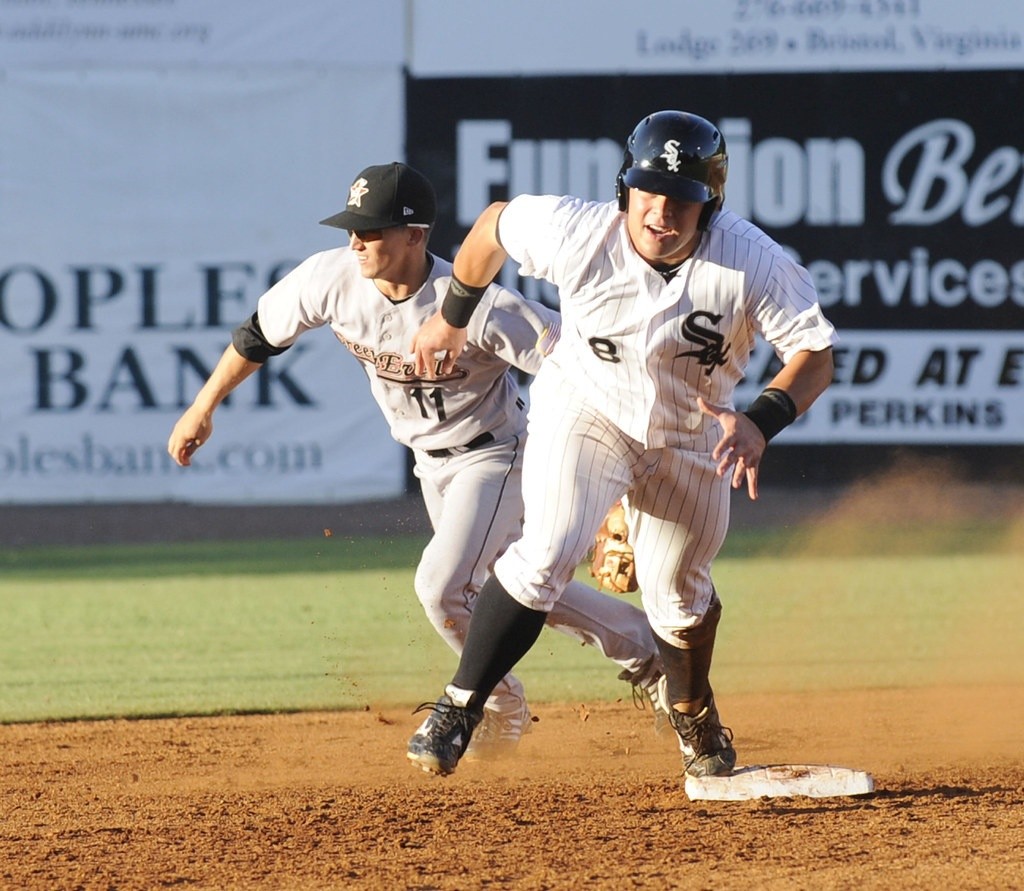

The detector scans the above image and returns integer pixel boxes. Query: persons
[403,109,839,777]
[169,163,681,766]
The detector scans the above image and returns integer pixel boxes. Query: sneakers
[464,697,532,762]
[618,647,671,734]
[657,674,737,780]
[406,683,484,778]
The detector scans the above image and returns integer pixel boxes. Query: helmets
[615,111,728,230]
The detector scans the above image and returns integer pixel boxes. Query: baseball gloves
[586,499,639,593]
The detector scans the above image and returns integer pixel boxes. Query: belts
[424,397,525,457]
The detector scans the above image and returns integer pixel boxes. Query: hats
[319,162,439,231]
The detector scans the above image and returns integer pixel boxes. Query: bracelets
[741,388,798,447]
[440,270,488,330]
